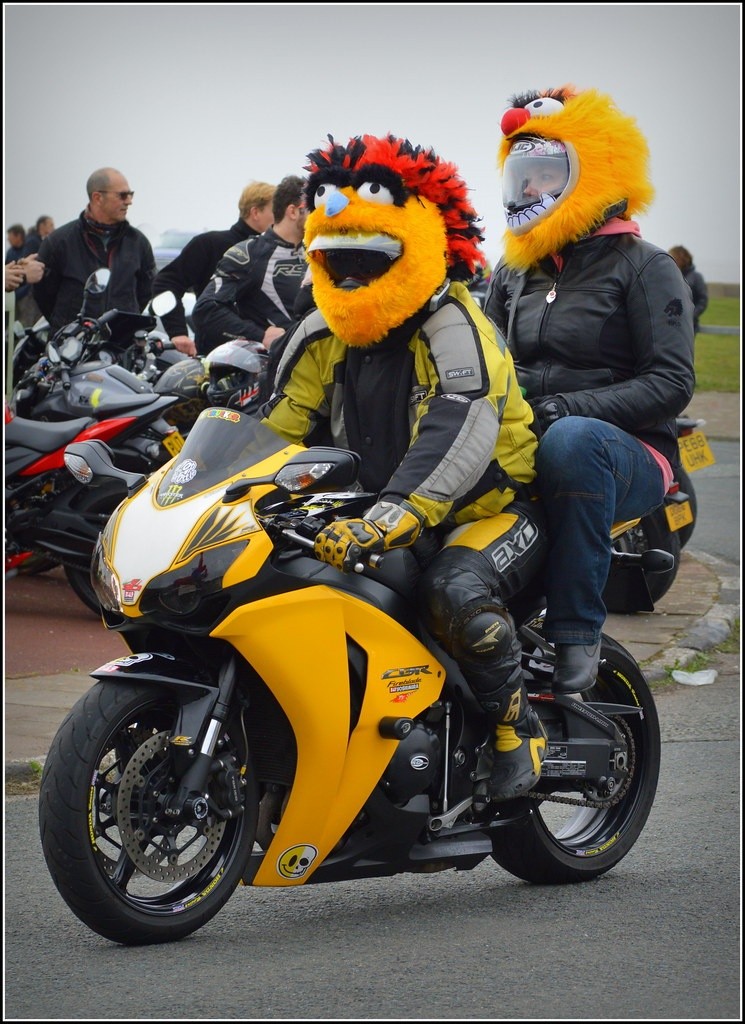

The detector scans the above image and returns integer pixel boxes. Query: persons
[6,167,710,331]
[482,83,696,694]
[225,131,548,804]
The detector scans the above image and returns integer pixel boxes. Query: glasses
[93,188,135,200]
[318,249,392,285]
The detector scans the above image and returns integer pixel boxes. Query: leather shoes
[551,638,604,698]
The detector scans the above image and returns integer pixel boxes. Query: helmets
[205,338,270,412]
[499,133,569,216]
[304,227,403,293]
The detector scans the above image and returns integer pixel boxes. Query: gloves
[524,393,570,435]
[314,500,422,576]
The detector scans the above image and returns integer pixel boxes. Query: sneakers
[485,721,549,803]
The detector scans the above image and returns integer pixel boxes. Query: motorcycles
[39,406,661,944]
[3,270,716,618]
